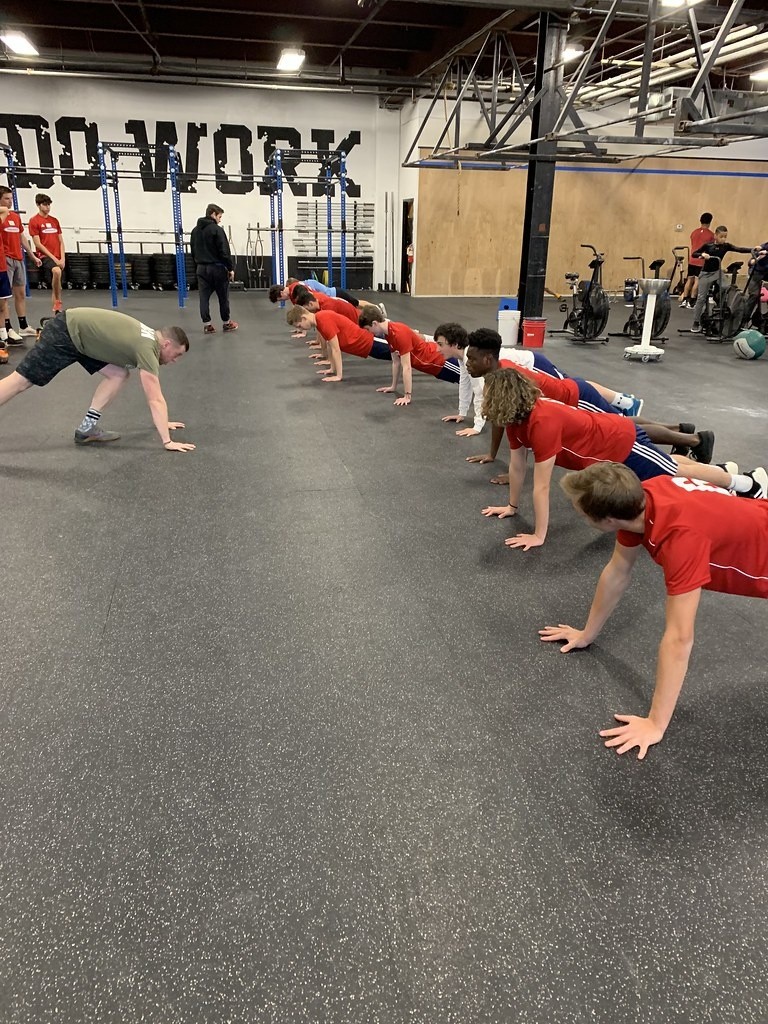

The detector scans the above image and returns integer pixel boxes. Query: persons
[538,461,768,759]
[691,225,760,330]
[269,277,436,381]
[743,243,768,324]
[465,327,715,484]
[0,185,65,348]
[190,204,239,334]
[434,322,644,438]
[0,307,196,453]
[358,305,460,406]
[481,367,768,551]
[679,212,715,309]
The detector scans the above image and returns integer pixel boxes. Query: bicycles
[677,248,760,341]
[674,246,691,296]
[608,246,684,339]
[548,242,609,339]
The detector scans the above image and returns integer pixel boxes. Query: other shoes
[377,303,387,319]
[741,322,758,330]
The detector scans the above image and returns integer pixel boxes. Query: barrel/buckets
[520,317,546,348]
[497,310,521,347]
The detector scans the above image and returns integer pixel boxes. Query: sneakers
[670,423,696,456]
[689,431,715,463]
[616,393,644,417]
[53,300,62,312]
[678,300,686,308]
[7,328,22,341]
[19,325,41,336]
[74,424,120,443]
[3,336,23,347]
[0,342,5,349]
[739,468,767,499]
[0,349,10,364]
[716,462,738,498]
[224,321,238,331]
[686,299,696,309]
[691,320,700,332]
[204,325,216,333]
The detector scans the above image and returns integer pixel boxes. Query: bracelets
[404,392,412,394]
[508,502,518,509]
[164,441,171,444]
